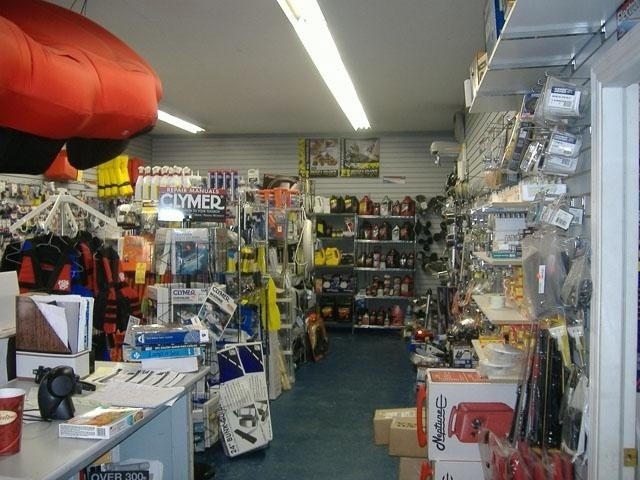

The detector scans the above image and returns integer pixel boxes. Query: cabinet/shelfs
[470,250,538,382]
[308,212,355,336]
[352,212,417,335]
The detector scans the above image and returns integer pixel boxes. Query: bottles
[359,220,413,240]
[337,196,345,212]
[359,246,415,268]
[374,202,380,214]
[332,228,343,237]
[394,200,400,215]
[357,274,411,328]
[133,165,188,200]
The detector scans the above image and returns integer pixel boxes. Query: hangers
[3,209,118,274]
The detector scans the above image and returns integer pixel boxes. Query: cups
[0,387,26,455]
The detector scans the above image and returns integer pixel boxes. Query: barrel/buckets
[364,220,415,241]
[400,275,414,297]
[389,305,404,325]
[318,295,335,320]
[386,249,400,267]
[335,295,352,322]
[248,166,260,187]
[326,246,341,267]
[314,194,415,217]
[314,248,325,266]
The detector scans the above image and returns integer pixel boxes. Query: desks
[0,360,211,480]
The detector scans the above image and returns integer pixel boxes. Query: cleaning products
[134,166,194,202]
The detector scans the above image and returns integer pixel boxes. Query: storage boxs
[398,457,432,480]
[416,367,524,462]
[419,461,486,480]
[372,406,426,446]
[388,418,428,458]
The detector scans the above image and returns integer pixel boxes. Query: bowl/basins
[482,342,524,378]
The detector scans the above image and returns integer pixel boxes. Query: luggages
[445,399,517,447]
